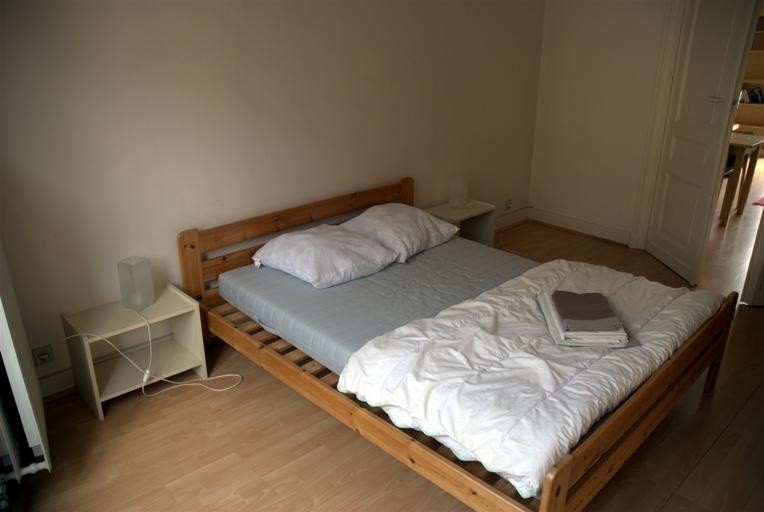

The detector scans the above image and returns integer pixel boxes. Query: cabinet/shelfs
[735,10,764,161]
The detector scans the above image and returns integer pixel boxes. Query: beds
[173,176,741,512]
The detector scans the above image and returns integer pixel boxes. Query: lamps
[118,256,154,312]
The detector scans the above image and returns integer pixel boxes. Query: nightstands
[57,279,209,423]
[424,195,497,247]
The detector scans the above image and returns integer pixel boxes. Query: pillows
[250,203,461,290]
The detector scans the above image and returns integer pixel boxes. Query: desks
[715,131,764,230]
